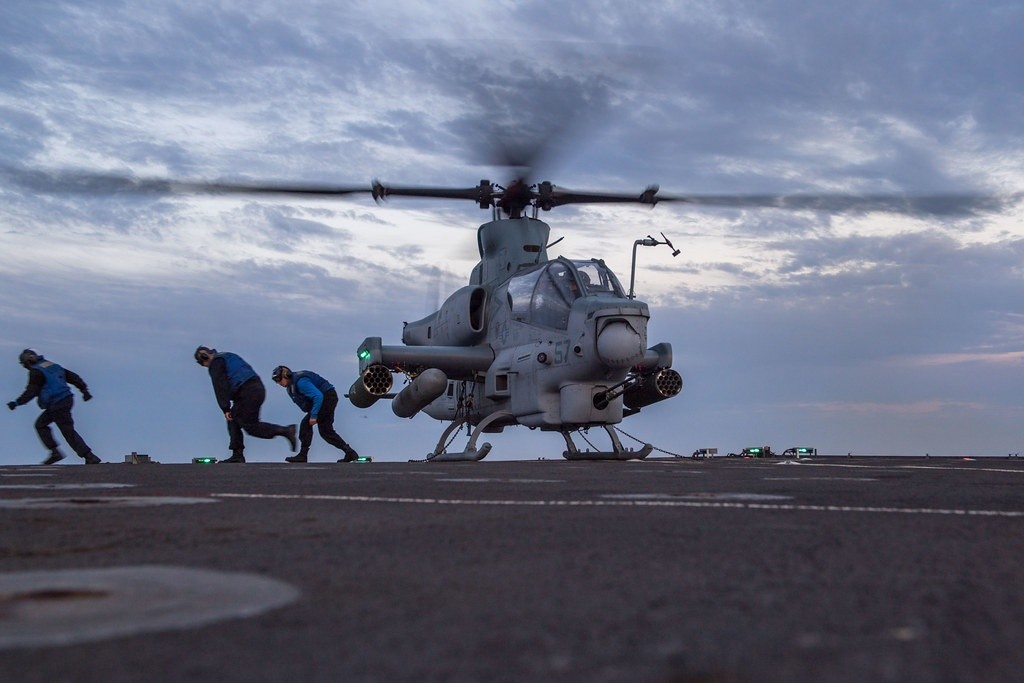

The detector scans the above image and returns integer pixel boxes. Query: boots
[285,446,310,462]
[337,443,359,462]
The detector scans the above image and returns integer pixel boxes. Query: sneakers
[45,451,62,464]
[285,424,297,452]
[218,454,246,463]
[85,454,101,464]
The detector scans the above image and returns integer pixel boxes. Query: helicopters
[210,141,684,461]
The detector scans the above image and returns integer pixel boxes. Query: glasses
[272,375,283,383]
[195,353,209,366]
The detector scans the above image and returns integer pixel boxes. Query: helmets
[18,348,38,363]
[270,365,292,379]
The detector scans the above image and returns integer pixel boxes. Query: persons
[7,347,103,466]
[564,270,591,301]
[272,364,360,464]
[194,345,297,464]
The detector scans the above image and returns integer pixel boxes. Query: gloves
[6,401,16,410]
[82,391,93,401]
